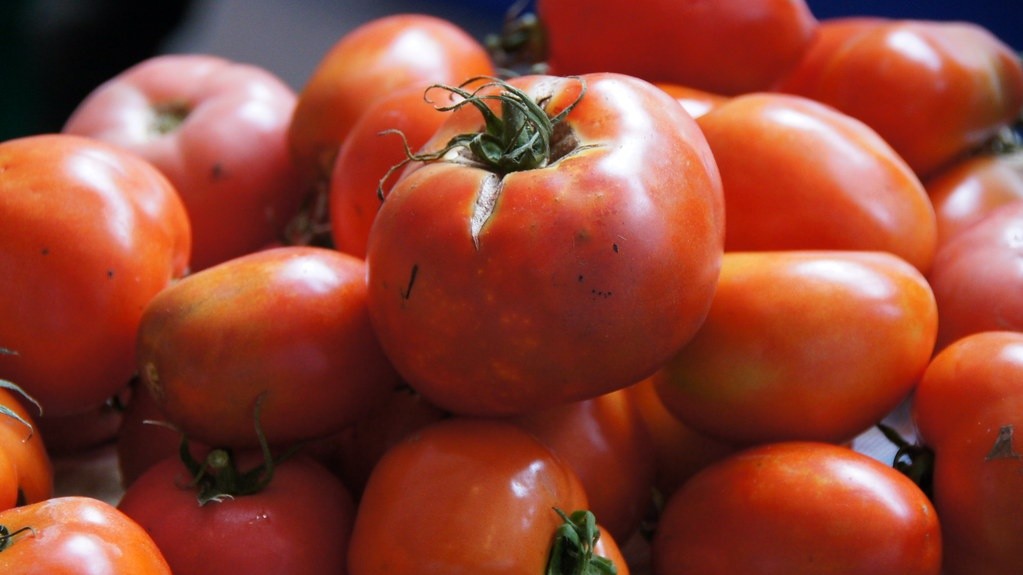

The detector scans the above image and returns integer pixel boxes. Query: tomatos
[3,1,1023,575]
